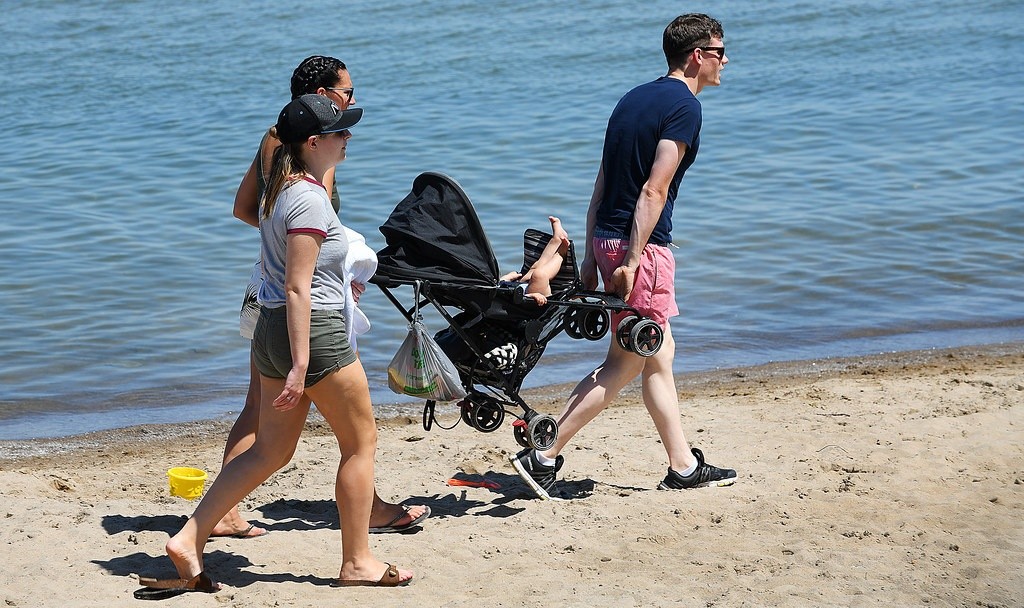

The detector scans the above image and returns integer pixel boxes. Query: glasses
[685,47,724,60]
[324,87,354,101]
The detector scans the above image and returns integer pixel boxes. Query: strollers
[367,173,663,451]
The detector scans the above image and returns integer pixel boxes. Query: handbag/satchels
[386,280,468,401]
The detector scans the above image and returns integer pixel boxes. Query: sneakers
[659,447,738,491]
[508,446,573,501]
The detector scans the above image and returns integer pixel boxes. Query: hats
[275,94,363,145]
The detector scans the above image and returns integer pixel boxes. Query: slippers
[140,571,222,593]
[333,562,413,587]
[208,524,269,539]
[369,504,431,533]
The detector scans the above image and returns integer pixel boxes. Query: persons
[499,215,570,306]
[138,93,414,592]
[509,12,736,501]
[211,54,431,540]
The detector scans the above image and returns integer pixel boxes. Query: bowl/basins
[167,467,208,501]
